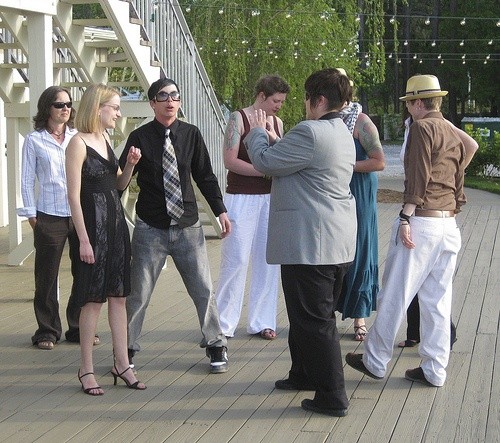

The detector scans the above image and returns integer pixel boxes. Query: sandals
[37,337,55,349]
[93,334,101,345]
[259,328,276,339]
[354,325,368,340]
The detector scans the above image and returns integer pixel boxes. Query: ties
[163,128,184,221]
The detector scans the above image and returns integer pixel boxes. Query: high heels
[110,364,145,390]
[78,367,104,395]
[398,339,420,347]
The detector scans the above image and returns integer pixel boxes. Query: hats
[335,68,353,87]
[399,75,448,101]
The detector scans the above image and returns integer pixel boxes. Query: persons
[61,86,147,397]
[14,86,103,351]
[344,73,470,386]
[206,75,289,339]
[397,101,480,352]
[112,77,234,370]
[331,66,386,338]
[242,67,357,417]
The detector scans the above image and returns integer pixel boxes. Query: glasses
[51,101,72,108]
[101,103,120,110]
[154,92,180,102]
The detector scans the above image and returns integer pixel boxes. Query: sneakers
[207,347,229,373]
[346,352,384,379]
[405,367,432,386]
[128,358,135,368]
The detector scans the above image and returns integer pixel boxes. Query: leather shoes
[301,399,348,416]
[275,379,316,390]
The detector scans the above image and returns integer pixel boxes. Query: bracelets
[398,209,410,225]
